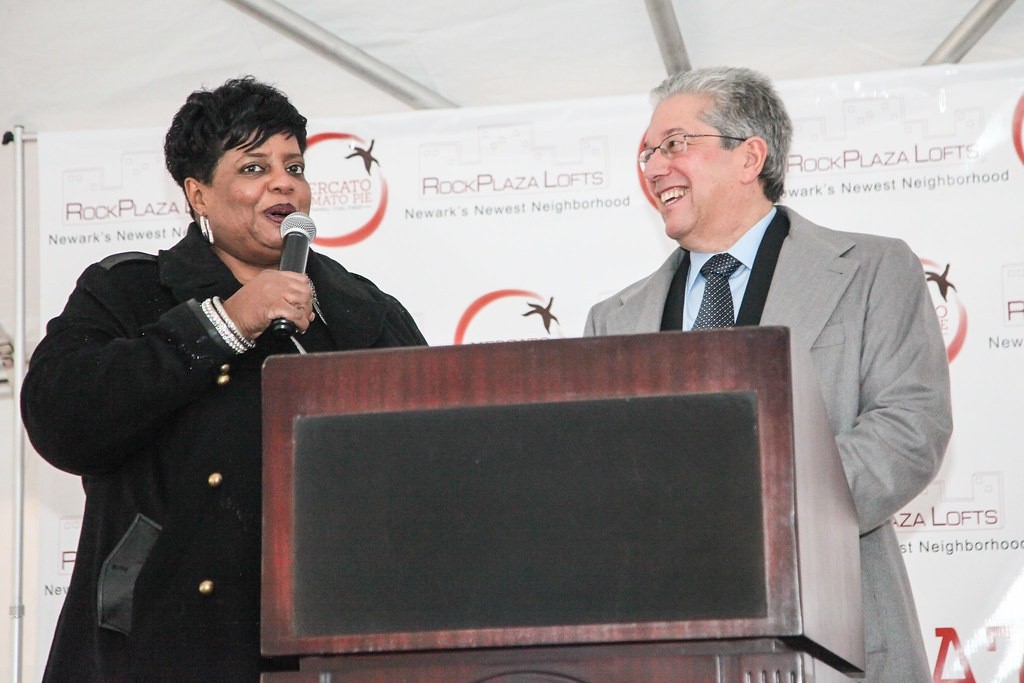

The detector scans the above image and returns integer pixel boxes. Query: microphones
[271,211,317,338]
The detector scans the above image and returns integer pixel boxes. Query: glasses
[638,132,746,174]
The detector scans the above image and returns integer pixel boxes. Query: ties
[691,254,743,330]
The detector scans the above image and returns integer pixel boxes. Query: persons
[20,75,429,683]
[583,67,952,683]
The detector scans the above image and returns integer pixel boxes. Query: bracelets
[201,297,256,354]
